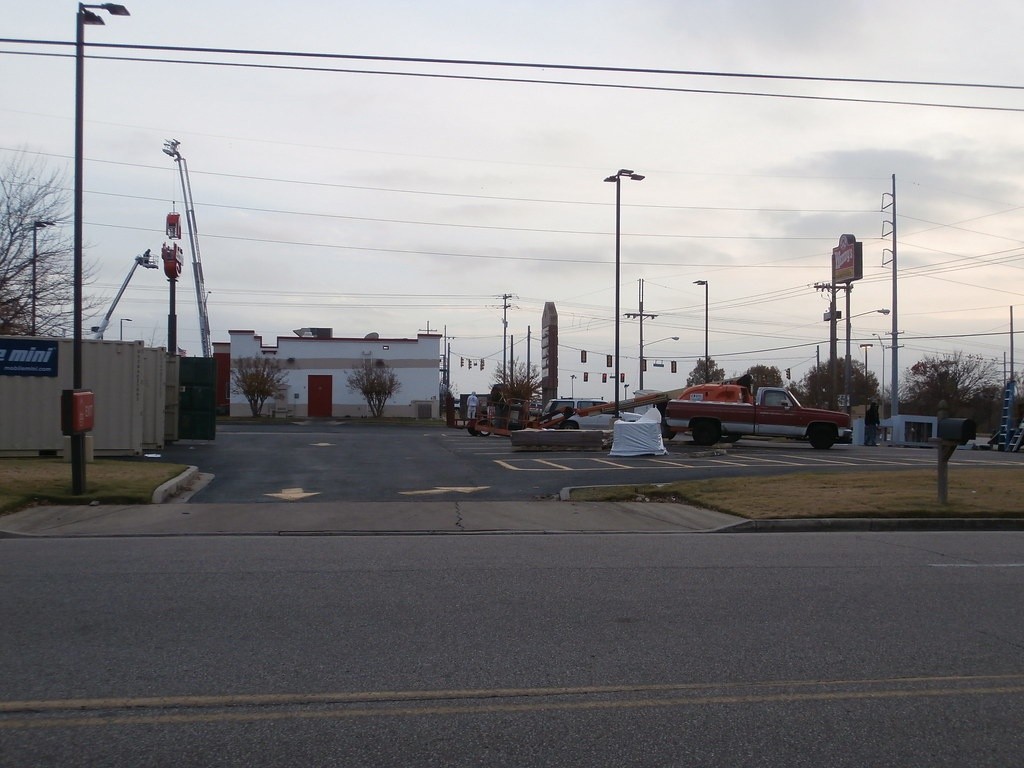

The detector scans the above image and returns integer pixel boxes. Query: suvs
[541,398,643,432]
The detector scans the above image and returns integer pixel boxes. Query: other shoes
[872,444,878,447]
[864,442,869,446]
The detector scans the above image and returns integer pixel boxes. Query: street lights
[872,333,885,439]
[828,309,890,409]
[624,385,629,400]
[860,344,873,416]
[640,337,680,389]
[30,220,56,335]
[570,374,576,398]
[120,319,132,339]
[603,169,645,418]
[72,1,132,495]
[693,280,708,385]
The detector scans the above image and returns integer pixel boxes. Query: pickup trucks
[664,387,853,450]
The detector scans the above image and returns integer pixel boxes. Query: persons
[143,249,151,264]
[163,242,170,250]
[864,403,880,446]
[467,392,478,419]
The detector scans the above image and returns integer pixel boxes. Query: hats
[871,402,877,407]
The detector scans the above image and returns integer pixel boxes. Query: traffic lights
[468,359,472,369]
[603,374,606,383]
[461,358,464,367]
[584,372,588,382]
[621,373,624,383]
[786,368,790,379]
[481,360,484,371]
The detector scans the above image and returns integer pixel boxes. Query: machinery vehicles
[162,139,213,358]
[92,253,160,339]
